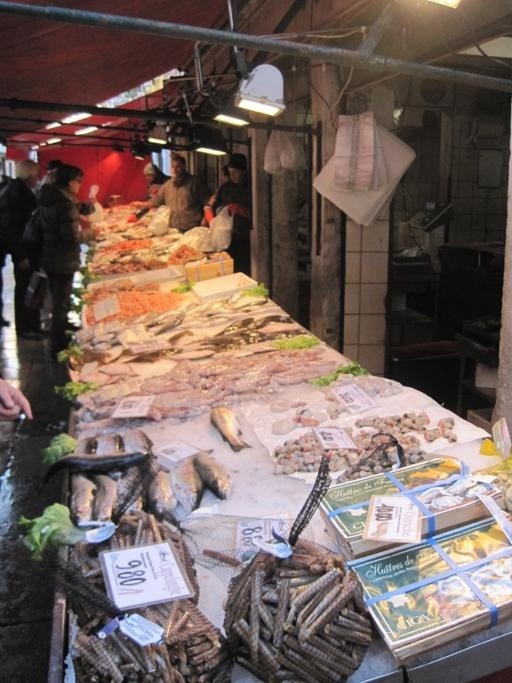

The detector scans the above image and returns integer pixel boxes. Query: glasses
[73,178,83,184]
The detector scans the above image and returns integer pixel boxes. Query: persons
[0,157,95,358]
[143,162,171,200]
[132,154,209,234]
[203,154,250,273]
[0,377,34,424]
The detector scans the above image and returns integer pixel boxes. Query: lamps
[44,60,285,159]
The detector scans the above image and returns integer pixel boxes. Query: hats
[44,160,64,169]
[224,153,247,168]
[143,162,160,175]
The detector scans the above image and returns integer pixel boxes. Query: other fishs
[210,406,253,452]
[68,288,339,438]
[67,428,232,526]
[40,452,151,485]
